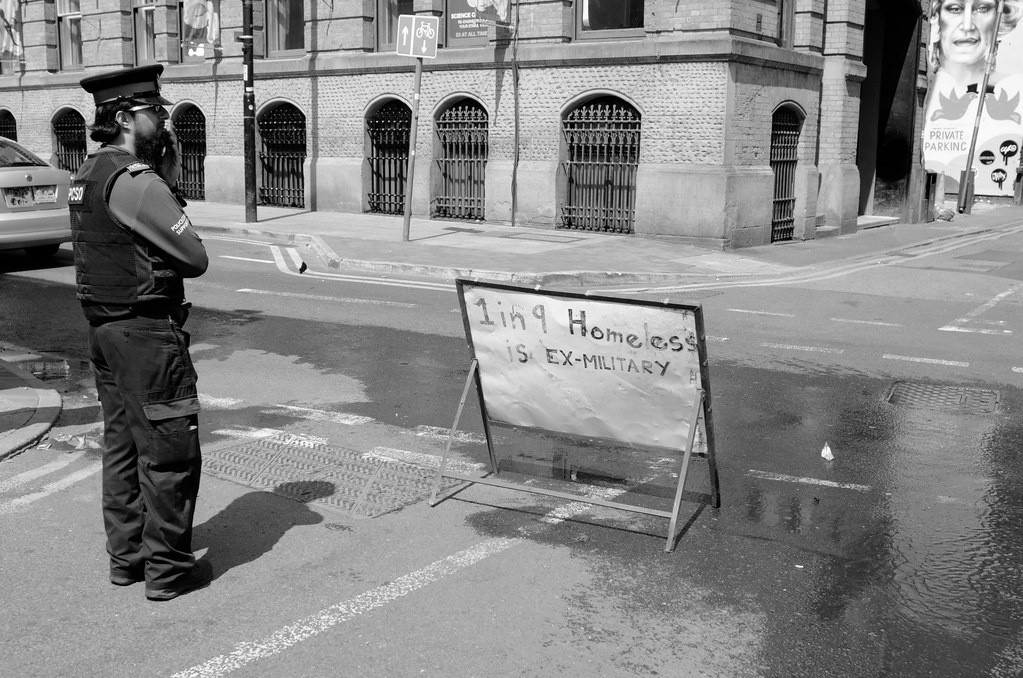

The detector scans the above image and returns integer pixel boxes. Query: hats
[79,64,173,106]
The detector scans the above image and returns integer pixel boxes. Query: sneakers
[145,558,212,600]
[109,574,134,585]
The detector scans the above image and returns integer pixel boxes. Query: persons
[62,63,213,600]
[931,0,1022,95]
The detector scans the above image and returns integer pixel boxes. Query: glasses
[126,103,160,112]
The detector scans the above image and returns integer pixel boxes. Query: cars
[0,137,76,250]
